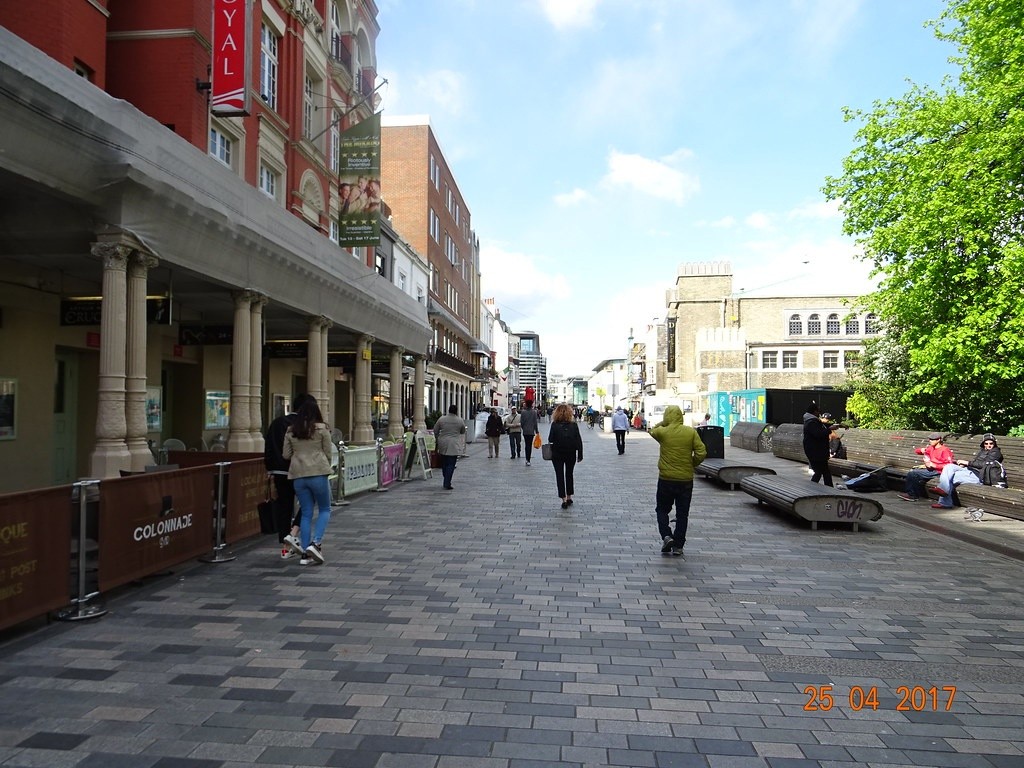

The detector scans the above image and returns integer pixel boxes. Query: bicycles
[587,415,594,429]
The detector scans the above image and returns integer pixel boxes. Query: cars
[476,406,511,434]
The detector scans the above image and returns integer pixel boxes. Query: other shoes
[897,493,919,501]
[511,456,515,459]
[526,460,530,466]
[517,452,520,458]
[929,488,948,497]
[618,451,624,455]
[496,456,498,458]
[567,499,573,505]
[284,535,306,555]
[281,548,298,558]
[562,502,567,509]
[488,456,493,458]
[306,541,324,562]
[443,485,453,489]
[932,504,952,509]
[300,553,313,564]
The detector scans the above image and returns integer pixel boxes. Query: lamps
[453,262,463,268]
[370,91,382,109]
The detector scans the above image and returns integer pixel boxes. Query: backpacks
[979,460,1008,488]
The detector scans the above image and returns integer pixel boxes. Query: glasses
[984,443,993,445]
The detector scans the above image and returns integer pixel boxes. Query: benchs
[741,475,884,532]
[825,428,955,483]
[917,433,1024,521]
[694,458,778,492]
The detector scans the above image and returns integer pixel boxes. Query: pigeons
[840,472,851,481]
[963,506,984,522]
[836,482,847,491]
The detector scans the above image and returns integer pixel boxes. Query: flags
[499,367,512,381]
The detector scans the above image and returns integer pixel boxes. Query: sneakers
[673,547,683,554]
[661,536,674,552]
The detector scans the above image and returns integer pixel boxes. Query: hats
[490,408,498,413]
[980,433,996,448]
[512,406,516,409]
[927,434,940,439]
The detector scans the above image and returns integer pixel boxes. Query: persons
[339,175,381,213]
[929,433,1004,508]
[548,404,583,509]
[803,400,849,488]
[485,406,522,459]
[623,407,644,430]
[897,432,952,501]
[700,413,712,426]
[571,404,595,429]
[265,394,332,565]
[434,404,465,489]
[612,406,630,455]
[536,403,556,424]
[649,404,706,556]
[521,401,539,465]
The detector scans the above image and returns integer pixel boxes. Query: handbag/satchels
[258,475,279,534]
[507,428,510,435]
[542,442,553,460]
[534,433,542,449]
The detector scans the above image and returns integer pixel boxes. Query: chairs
[328,432,437,501]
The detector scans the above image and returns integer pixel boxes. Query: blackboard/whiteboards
[404,433,432,473]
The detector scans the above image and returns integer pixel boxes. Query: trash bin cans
[696,426,724,458]
